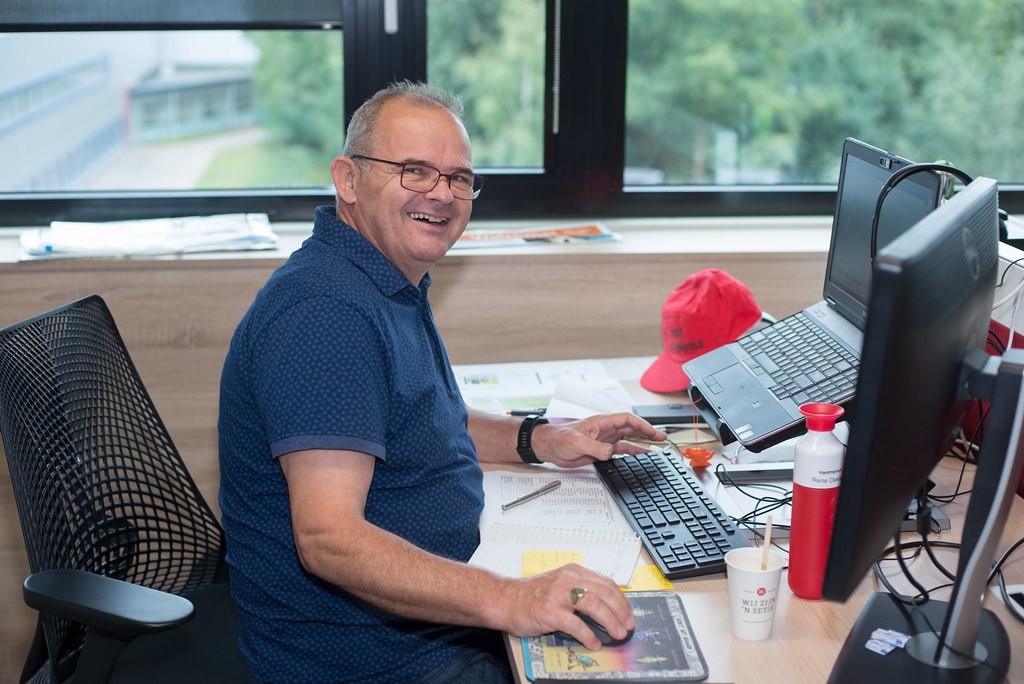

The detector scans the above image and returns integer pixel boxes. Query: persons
[216,81,668,684]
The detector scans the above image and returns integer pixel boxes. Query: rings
[570,587,588,607]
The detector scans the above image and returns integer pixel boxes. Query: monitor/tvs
[820,176,1024,683]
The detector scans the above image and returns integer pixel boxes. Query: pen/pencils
[505,410,545,416]
[502,480,561,511]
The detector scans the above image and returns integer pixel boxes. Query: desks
[448,356,1024,684]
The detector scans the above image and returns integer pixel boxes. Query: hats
[642,269,761,392]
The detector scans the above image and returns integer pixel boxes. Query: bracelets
[515,414,550,464]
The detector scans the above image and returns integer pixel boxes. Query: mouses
[554,610,635,647]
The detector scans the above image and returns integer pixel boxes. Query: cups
[724,547,785,641]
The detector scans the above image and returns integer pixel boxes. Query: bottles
[788,403,847,600]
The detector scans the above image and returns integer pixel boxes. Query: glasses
[349,154,488,201]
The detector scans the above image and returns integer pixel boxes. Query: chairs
[0,293,261,684]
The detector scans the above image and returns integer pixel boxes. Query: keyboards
[593,448,755,580]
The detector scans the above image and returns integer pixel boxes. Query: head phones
[870,163,1009,266]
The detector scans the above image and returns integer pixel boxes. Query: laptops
[681,136,942,454]
